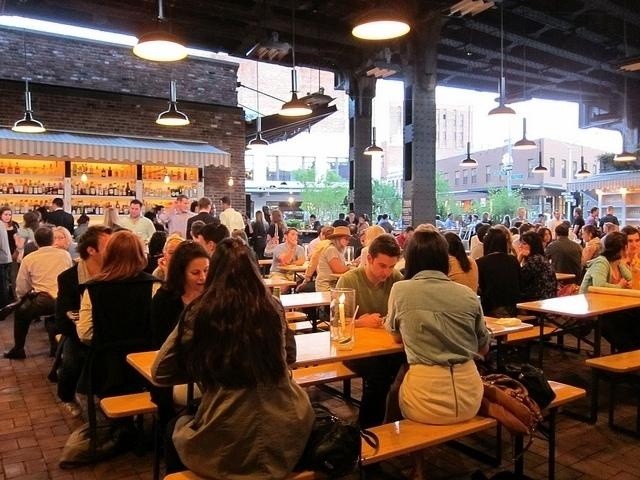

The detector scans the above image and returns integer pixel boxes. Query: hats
[326,225,352,239]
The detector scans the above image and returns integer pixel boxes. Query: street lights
[501,152,513,199]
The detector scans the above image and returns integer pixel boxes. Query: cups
[461,240,470,252]
[343,246,361,265]
[329,286,358,352]
[288,288,295,294]
[302,242,311,262]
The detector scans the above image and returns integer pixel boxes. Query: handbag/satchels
[263,222,280,258]
[480,362,556,440]
[292,402,379,477]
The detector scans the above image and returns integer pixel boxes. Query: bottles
[273,287,281,298]
[1,161,198,215]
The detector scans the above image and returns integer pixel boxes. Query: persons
[0,195,640,480]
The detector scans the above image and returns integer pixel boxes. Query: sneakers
[2,347,27,360]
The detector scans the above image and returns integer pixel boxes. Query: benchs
[163,380,586,480]
[100,362,361,480]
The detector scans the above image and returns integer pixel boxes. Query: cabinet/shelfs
[598,192,640,229]
[0,158,200,218]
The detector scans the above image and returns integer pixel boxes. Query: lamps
[461,0,640,176]
[164,168,169,183]
[81,171,87,180]
[227,171,234,186]
[12,26,46,134]
